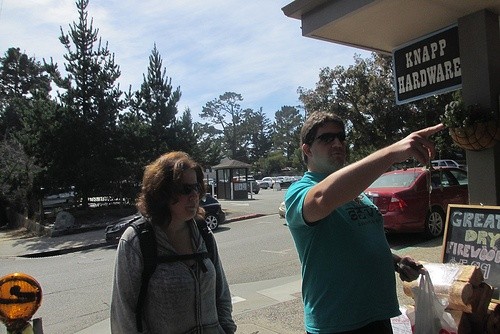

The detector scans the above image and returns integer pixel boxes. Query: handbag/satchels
[406,267,458,334]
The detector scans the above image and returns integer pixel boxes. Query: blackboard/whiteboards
[440,204,500,306]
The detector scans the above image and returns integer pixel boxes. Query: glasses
[313,133,345,144]
[172,183,199,194]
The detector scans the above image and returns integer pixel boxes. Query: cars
[417,160,461,167]
[204,175,303,194]
[104,194,226,246]
[361,167,469,240]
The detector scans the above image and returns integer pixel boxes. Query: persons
[283,111,444,334]
[110,151,237,334]
